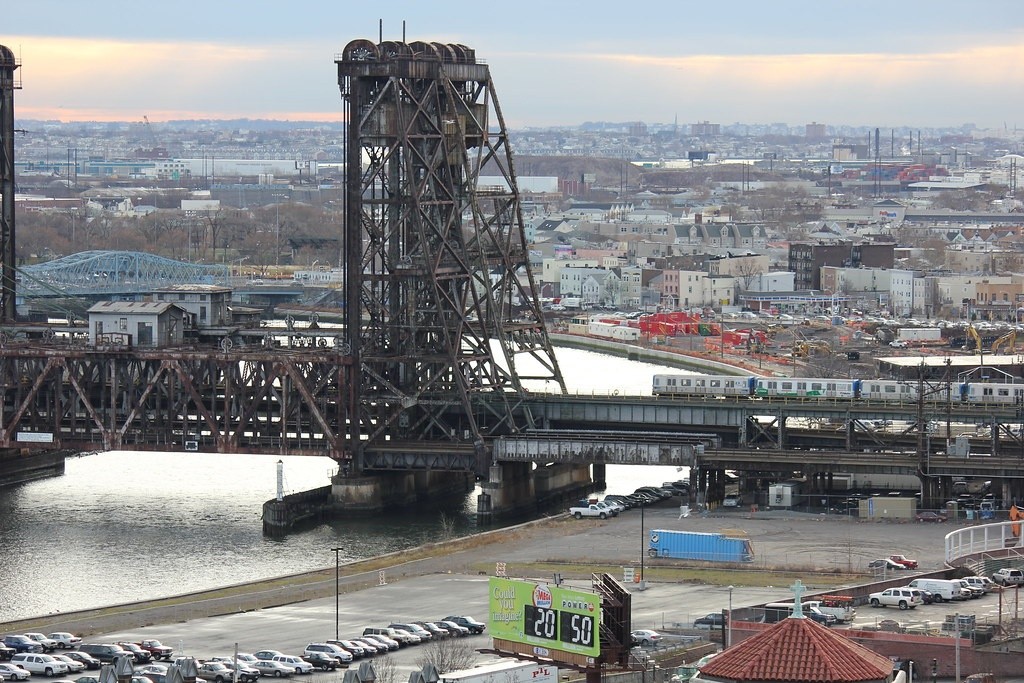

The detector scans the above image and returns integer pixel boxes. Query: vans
[810,607,837,628]
[903,579,959,604]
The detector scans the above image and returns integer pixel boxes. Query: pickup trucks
[804,600,856,624]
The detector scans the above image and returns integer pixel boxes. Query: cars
[953,481,995,512]
[0,615,486,683]
[869,558,907,572]
[901,585,933,605]
[630,629,664,647]
[542,294,1024,330]
[569,470,740,520]
[916,511,948,524]
[824,491,922,513]
[951,576,1000,599]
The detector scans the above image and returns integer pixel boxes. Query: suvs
[868,587,924,611]
[890,554,919,570]
[991,567,1024,588]
[693,611,723,630]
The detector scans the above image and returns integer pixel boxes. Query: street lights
[311,260,319,272]
[326,260,332,271]
[634,496,651,591]
[330,546,345,641]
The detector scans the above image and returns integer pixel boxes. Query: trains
[652,373,1023,407]
